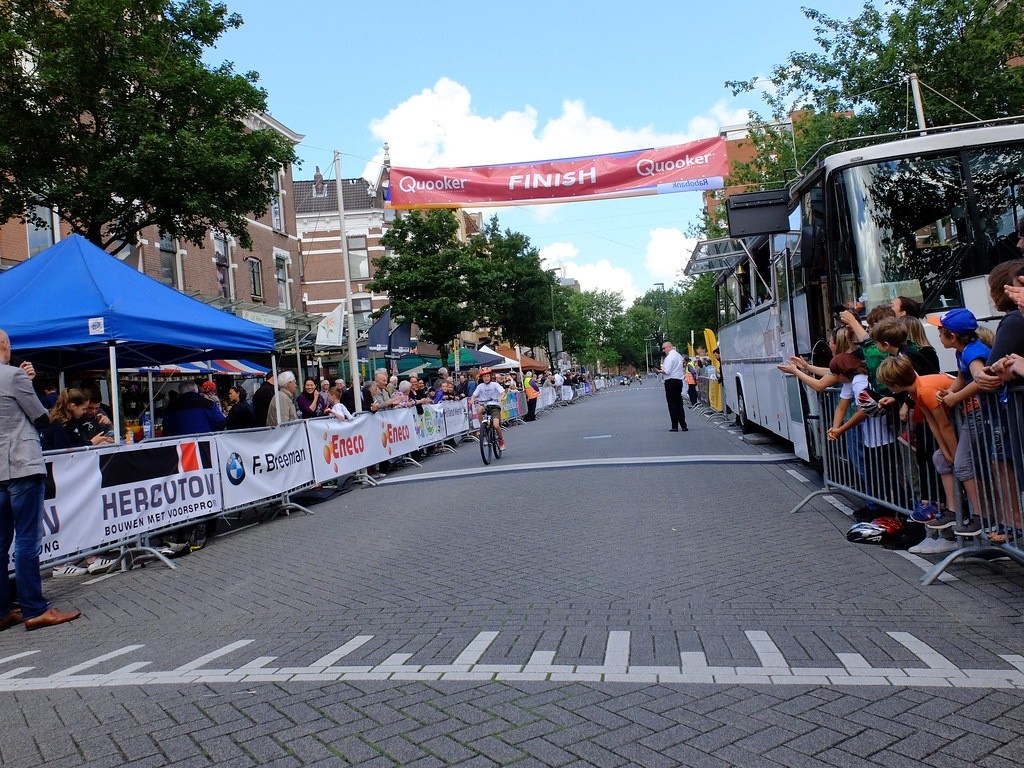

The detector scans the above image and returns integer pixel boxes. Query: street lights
[547,268,562,371]
[654,283,670,342]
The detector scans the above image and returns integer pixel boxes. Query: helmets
[856,387,892,418]
[846,522,886,544]
[870,516,904,535]
[202,380,216,392]
[479,367,492,375]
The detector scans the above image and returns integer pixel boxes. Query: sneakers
[87,557,120,575]
[52,562,87,579]
[953,515,998,536]
[908,537,936,554]
[921,537,959,554]
[927,510,970,529]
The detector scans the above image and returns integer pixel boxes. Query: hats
[926,308,980,334]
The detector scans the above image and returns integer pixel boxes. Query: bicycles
[468,398,504,465]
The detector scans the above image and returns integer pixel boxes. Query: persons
[652,341,689,432]
[0,324,82,632]
[684,347,733,415]
[40,367,643,579]
[778,217,1024,553]
[469,368,507,451]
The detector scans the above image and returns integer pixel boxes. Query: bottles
[125,431,134,445]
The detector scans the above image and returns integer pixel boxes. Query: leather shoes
[25,607,81,631]
[0,608,23,631]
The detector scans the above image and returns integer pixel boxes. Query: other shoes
[499,438,506,450]
[682,428,688,431]
[669,428,678,432]
[897,430,917,452]
[906,503,942,523]
[369,472,387,478]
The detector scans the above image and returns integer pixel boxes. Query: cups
[130,403,136,409]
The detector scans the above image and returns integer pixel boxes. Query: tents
[0,234,279,444]
[397,344,548,375]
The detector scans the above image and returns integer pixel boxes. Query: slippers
[988,527,1023,543]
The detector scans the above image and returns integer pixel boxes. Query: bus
[683,113,1024,462]
